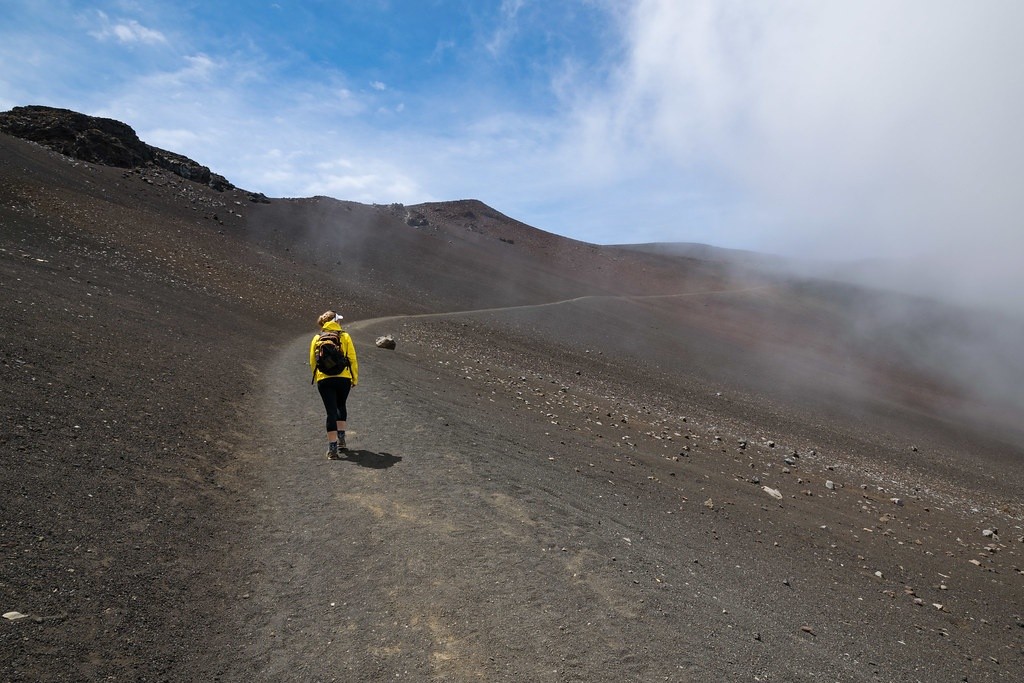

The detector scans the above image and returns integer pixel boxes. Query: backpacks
[317,331,346,375]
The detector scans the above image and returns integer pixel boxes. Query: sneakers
[338,442,346,449]
[326,451,338,460]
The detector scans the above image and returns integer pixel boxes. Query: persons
[311,312,357,459]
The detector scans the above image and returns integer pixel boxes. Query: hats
[329,313,344,322]
